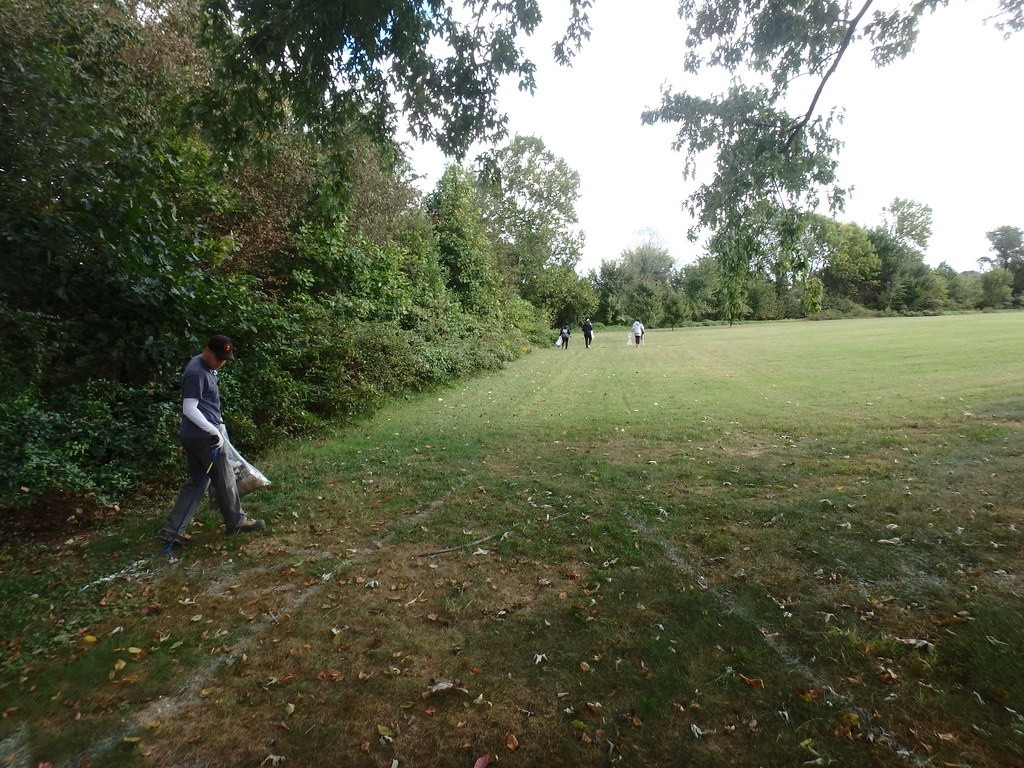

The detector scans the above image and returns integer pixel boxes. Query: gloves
[210,432,224,450]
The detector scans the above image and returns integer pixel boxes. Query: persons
[582,318,593,349]
[154,334,266,544]
[631,319,645,347]
[559,321,570,350]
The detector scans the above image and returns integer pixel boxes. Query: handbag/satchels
[627,332,633,345]
[556,336,562,346]
[591,330,595,340]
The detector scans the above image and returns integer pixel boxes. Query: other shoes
[588,345,591,349]
[636,345,639,347]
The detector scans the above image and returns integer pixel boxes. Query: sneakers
[226,517,265,532]
[158,528,193,545]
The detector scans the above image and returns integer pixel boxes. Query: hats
[208,335,235,361]
